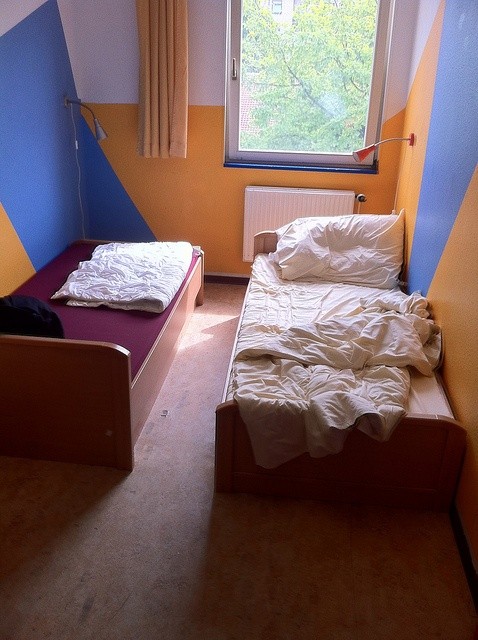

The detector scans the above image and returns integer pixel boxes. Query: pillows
[273,214,405,291]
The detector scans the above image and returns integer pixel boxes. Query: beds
[214,210,467,514]
[0,238,206,473]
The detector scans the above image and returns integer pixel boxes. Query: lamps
[352,133,415,162]
[65,100,110,144]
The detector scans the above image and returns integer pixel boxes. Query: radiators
[242,187,366,264]
